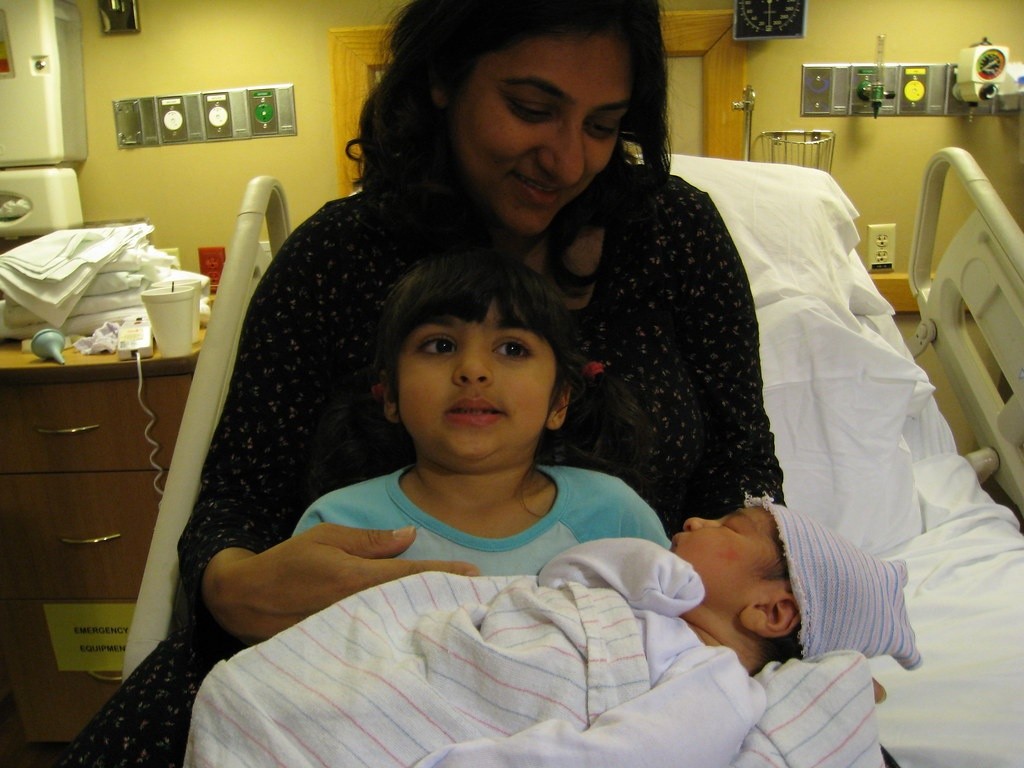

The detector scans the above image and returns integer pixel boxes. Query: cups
[142,286,196,355]
[151,280,200,342]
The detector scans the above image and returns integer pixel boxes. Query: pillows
[664,154,897,318]
[755,293,930,560]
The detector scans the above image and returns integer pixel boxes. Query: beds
[123,146,1024,768]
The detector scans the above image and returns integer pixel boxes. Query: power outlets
[867,222,897,274]
[198,247,226,292]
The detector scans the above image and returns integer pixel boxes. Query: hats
[744,491,922,671]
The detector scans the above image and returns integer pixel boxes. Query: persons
[69,4,891,768]
[184,495,920,768]
[292,259,674,582]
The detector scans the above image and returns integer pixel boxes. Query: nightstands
[0,293,217,743]
